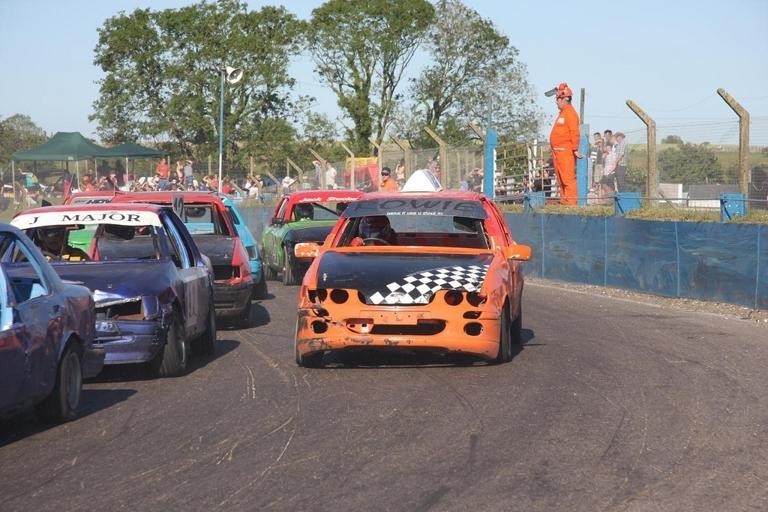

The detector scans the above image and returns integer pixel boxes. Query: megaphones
[224,65,244,84]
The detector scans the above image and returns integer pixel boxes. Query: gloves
[349,236,366,246]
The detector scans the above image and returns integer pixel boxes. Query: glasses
[381,171,389,176]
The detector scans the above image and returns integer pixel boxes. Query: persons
[45,229,88,260]
[460,126,630,208]
[348,214,391,247]
[154,158,171,191]
[0,175,5,211]
[176,159,185,185]
[325,162,338,190]
[74,170,265,197]
[544,83,582,208]
[17,164,41,208]
[380,166,398,192]
[178,160,194,184]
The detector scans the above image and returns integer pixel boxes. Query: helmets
[37,227,67,250]
[295,203,314,221]
[358,215,392,240]
